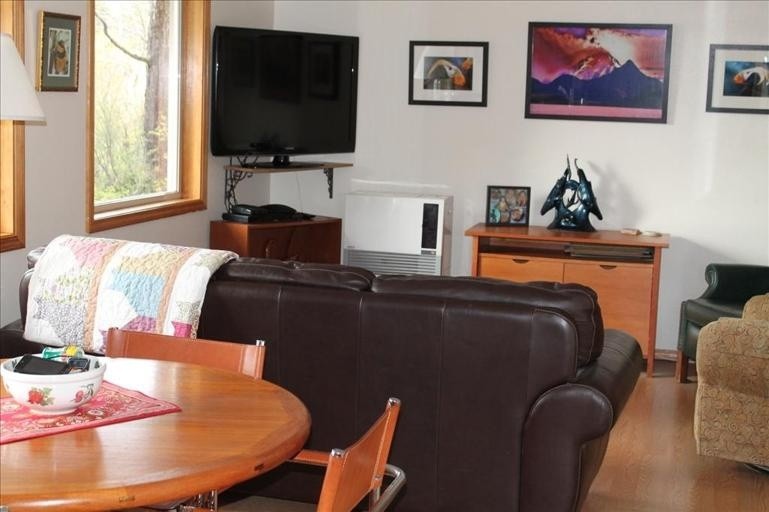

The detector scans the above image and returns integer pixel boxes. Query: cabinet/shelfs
[210,217,339,272]
[465,226,672,378]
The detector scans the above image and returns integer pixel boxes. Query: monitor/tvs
[210,26,360,170]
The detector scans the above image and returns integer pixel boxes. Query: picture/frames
[35,11,82,93]
[407,39,489,109]
[522,22,669,123]
[705,45,769,116]
[485,185,532,229]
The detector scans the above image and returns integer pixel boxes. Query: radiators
[339,183,453,280]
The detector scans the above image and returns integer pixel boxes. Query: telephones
[221,204,271,224]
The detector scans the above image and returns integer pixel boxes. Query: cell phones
[14,354,66,374]
[67,358,90,374]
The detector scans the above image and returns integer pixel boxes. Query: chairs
[96,330,272,384]
[205,392,405,511]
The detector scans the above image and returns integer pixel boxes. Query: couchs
[691,290,769,471]
[677,263,769,383]
[0,247,645,510]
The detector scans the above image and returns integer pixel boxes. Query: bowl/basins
[1,353,107,416]
